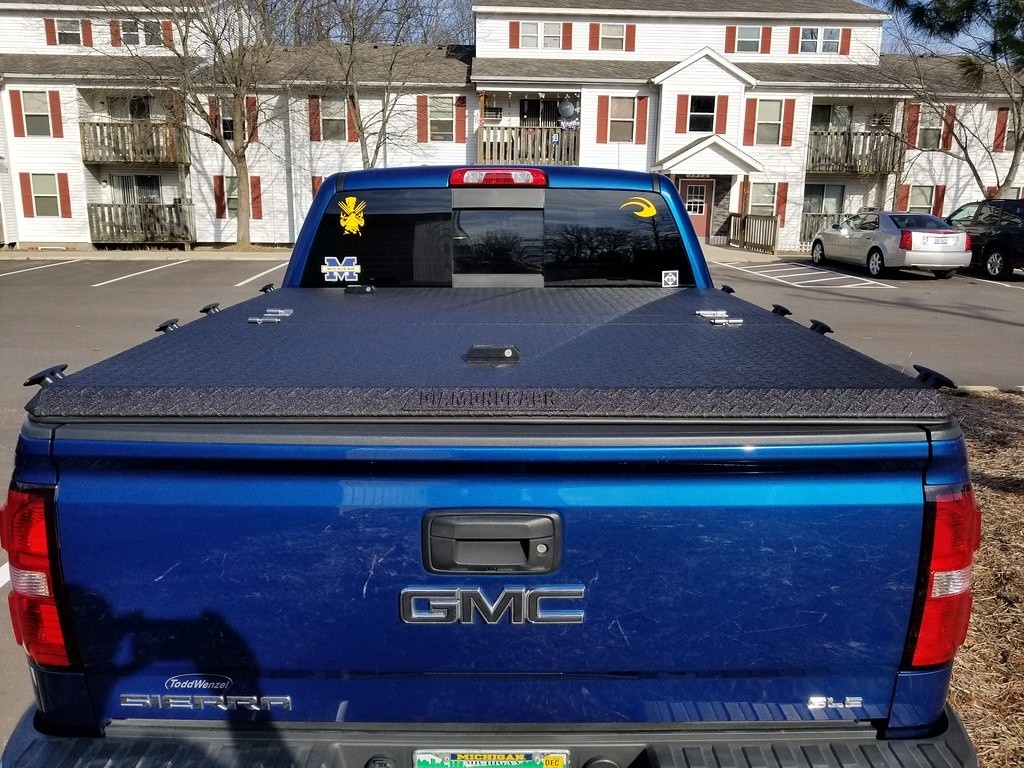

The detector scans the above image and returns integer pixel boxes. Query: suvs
[941,199,1024,280]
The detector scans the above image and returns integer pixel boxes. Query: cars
[809,210,972,280]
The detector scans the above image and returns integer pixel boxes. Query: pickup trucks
[0,163,984,768]
